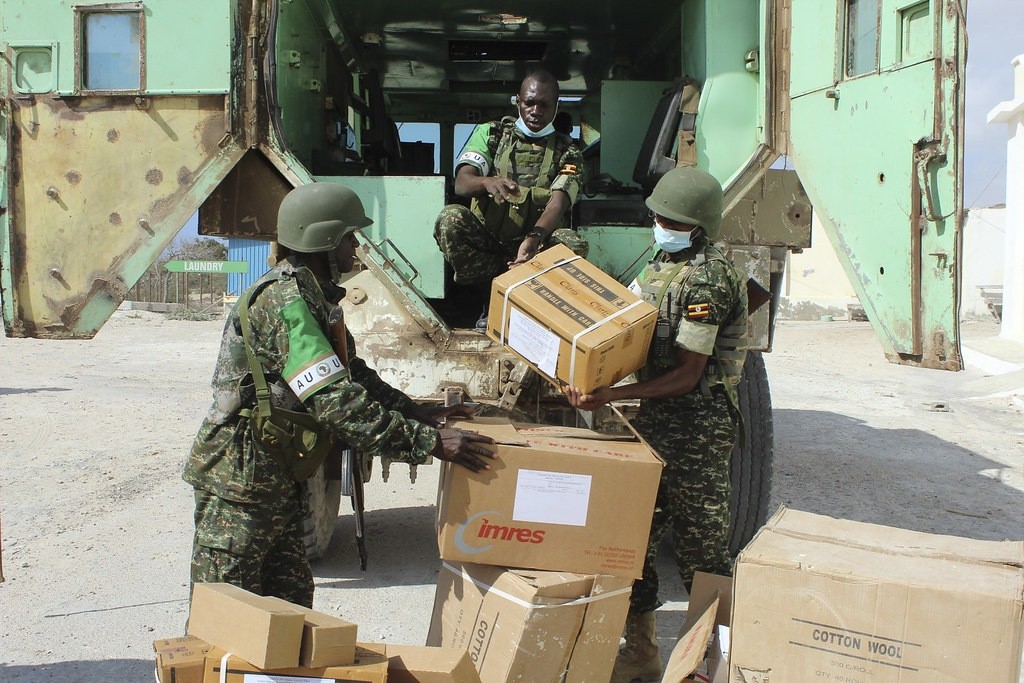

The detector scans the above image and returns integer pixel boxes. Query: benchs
[572,193,646,228]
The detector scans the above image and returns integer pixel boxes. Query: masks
[514,105,559,140]
[650,222,702,253]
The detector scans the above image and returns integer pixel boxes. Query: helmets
[275,181,375,253]
[644,164,724,250]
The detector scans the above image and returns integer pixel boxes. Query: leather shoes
[475,302,488,334]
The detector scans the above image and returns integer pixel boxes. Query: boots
[609,613,664,683]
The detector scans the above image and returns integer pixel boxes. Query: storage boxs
[415,243,1020,681]
[156,577,474,681]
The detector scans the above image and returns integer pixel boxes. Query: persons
[564,167,748,683]
[183,182,498,610]
[434,72,587,286]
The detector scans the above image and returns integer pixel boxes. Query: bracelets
[527,226,545,241]
